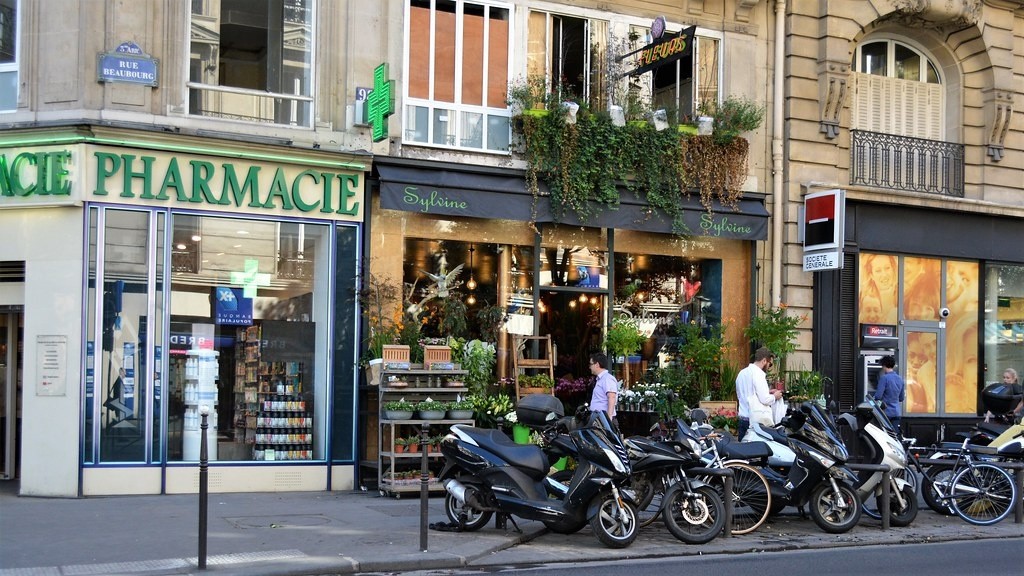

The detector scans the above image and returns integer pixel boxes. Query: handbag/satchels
[771,397,788,426]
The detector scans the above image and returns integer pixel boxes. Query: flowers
[555,376,595,394]
[617,380,666,404]
[481,394,544,449]
[395,438,406,445]
[666,315,737,396]
[707,405,739,430]
[789,396,808,402]
[742,300,810,358]
[417,334,516,394]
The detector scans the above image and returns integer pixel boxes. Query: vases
[629,403,639,411]
[552,456,568,471]
[641,401,653,412]
[617,402,627,411]
[512,424,530,445]
[762,356,774,381]
[715,428,739,436]
[700,395,712,402]
[794,402,803,410]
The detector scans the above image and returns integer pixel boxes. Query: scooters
[436,403,641,550]
[516,381,1024,545]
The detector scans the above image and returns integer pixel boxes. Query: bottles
[415,377,420,387]
[259,376,263,392]
[437,377,441,387]
[184,384,199,402]
[257,412,313,428]
[215,384,218,401]
[265,395,305,412]
[215,409,218,426]
[276,381,284,395]
[255,444,312,460]
[186,358,198,376]
[184,409,198,430]
[428,377,433,388]
[255,429,312,444]
[286,380,294,395]
[215,359,219,376]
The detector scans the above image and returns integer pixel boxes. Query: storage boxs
[366,412,401,462]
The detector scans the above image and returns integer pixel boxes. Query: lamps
[466,249,477,290]
[467,291,476,304]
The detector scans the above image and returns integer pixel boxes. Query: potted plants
[384,375,476,481]
[518,373,555,394]
[364,332,396,380]
[502,26,769,137]
[359,357,372,384]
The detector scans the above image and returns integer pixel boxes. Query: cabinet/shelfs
[173,325,314,460]
[358,385,436,491]
[379,369,475,499]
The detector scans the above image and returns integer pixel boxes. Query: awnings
[375,156,770,242]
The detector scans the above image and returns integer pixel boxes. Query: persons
[907,332,936,413]
[904,256,940,321]
[945,261,978,413]
[875,355,904,442]
[736,347,783,427]
[860,256,898,323]
[589,354,619,430]
[983,368,1024,423]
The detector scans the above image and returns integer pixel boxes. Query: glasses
[766,360,773,368]
[588,363,598,367]
